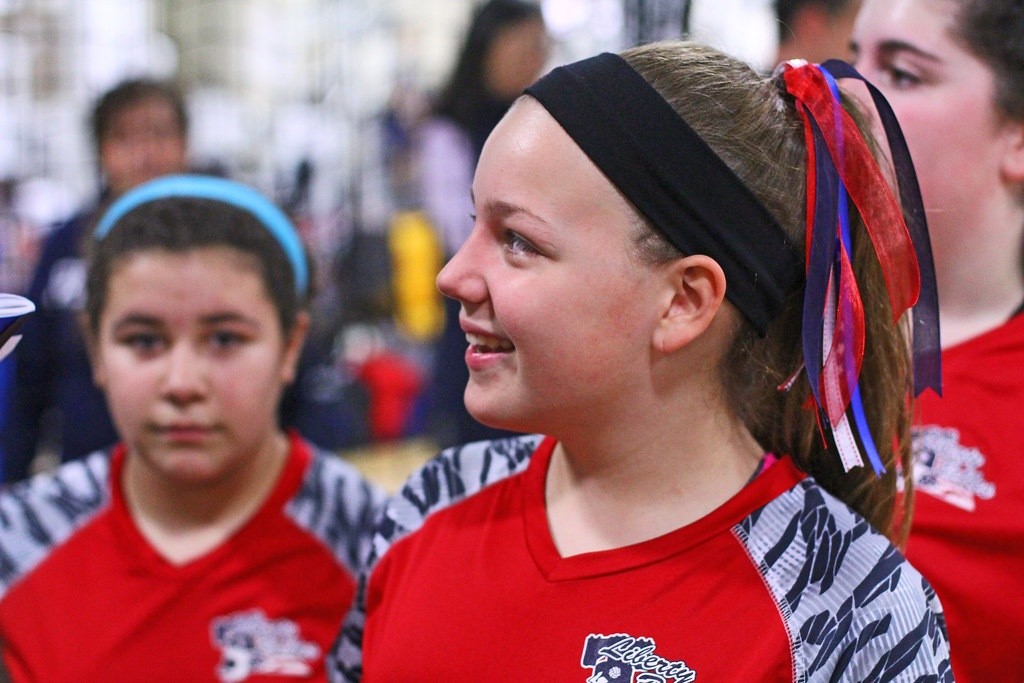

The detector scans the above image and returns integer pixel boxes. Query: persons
[362,41,960,682]
[0,0,1023,683]
